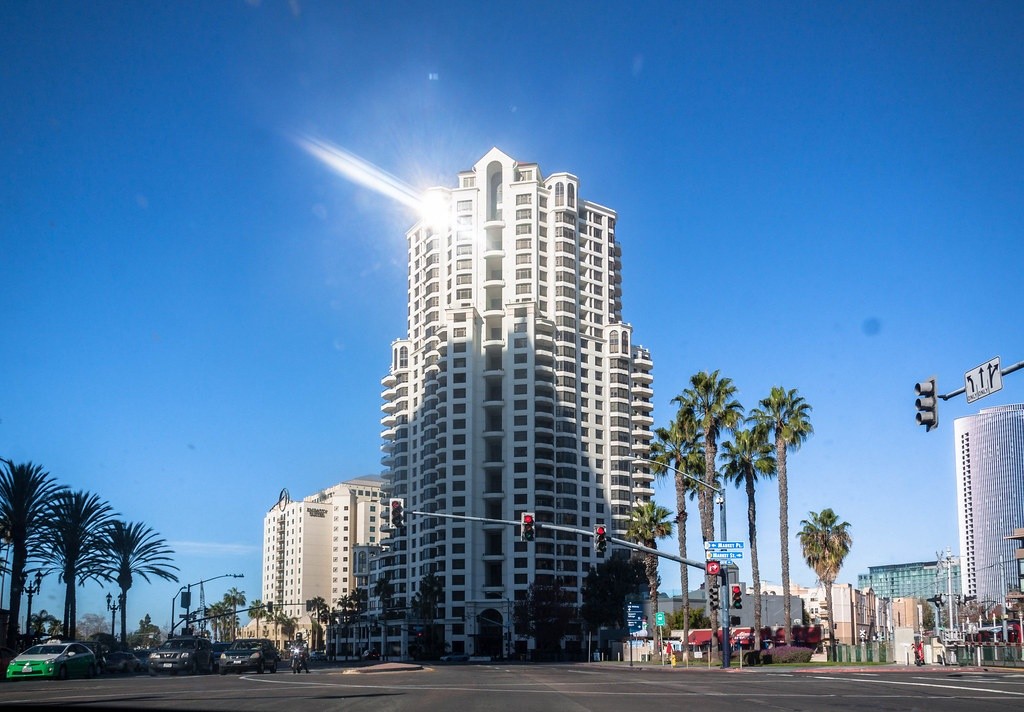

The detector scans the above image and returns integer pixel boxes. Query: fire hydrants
[670,654,678,668]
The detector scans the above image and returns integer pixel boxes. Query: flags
[666,641,673,653]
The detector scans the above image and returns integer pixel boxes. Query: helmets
[296,633,302,639]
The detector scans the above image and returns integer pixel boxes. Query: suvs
[147,637,215,677]
[440,651,470,662]
[362,650,380,661]
[220,638,277,674]
[309,651,328,661]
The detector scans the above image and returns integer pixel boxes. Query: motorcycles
[288,642,305,674]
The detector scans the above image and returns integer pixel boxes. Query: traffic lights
[927,595,946,607]
[709,585,719,609]
[520,512,537,542]
[389,499,405,530]
[729,584,743,610]
[594,524,607,553]
[915,375,938,432]
[956,593,974,605]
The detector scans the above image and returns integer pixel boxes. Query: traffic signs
[704,541,744,560]
[731,616,741,626]
[624,605,642,630]
[964,355,1005,403]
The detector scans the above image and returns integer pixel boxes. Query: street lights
[171,586,188,638]
[105,592,123,637]
[20,569,43,650]
[632,457,729,669]
[186,574,244,635]
[487,591,510,660]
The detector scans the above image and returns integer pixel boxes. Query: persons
[371,647,379,655]
[292,632,311,673]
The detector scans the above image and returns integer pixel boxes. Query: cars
[211,641,234,667]
[6,640,152,681]
